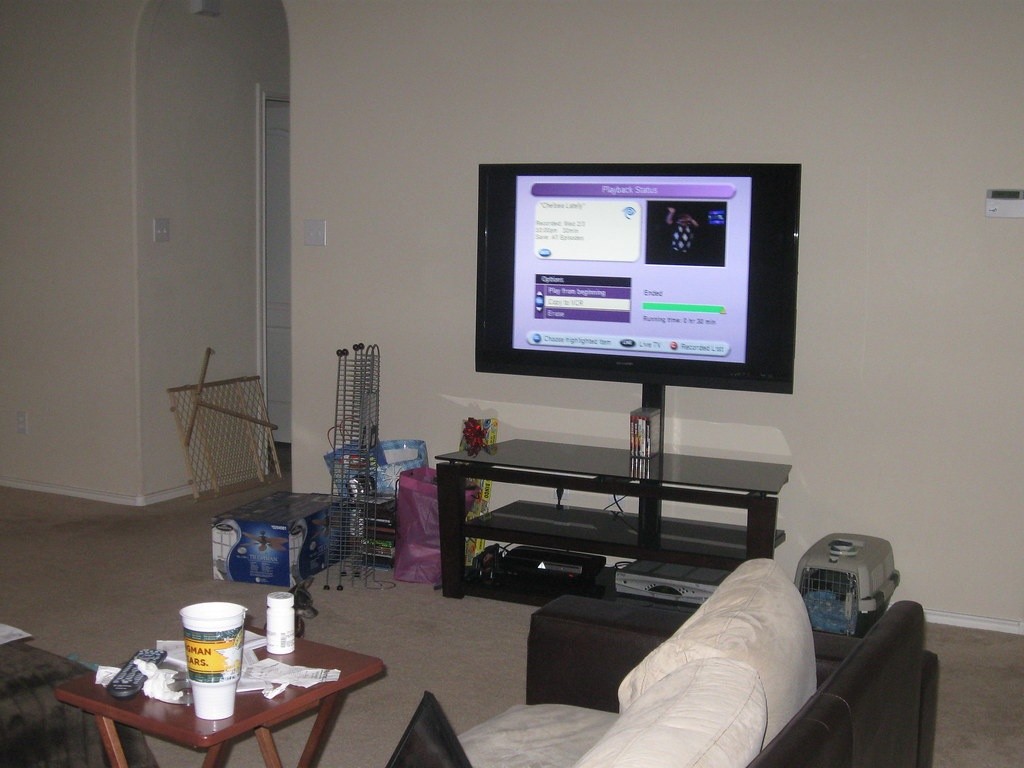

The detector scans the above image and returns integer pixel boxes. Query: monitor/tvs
[476,164,800,393]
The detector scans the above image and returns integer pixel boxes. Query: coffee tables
[55,625,382,768]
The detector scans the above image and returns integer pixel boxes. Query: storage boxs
[210,492,351,588]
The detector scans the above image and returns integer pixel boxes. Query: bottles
[265,590,296,655]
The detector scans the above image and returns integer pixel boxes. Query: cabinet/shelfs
[434,439,792,606]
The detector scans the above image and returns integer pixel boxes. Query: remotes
[107,648,168,699]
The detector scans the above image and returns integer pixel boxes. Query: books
[629,407,660,458]
[629,456,658,480]
[363,516,395,555]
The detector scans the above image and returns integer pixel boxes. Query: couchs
[386,559,940,768]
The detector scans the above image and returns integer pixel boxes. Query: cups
[179,601,248,721]
